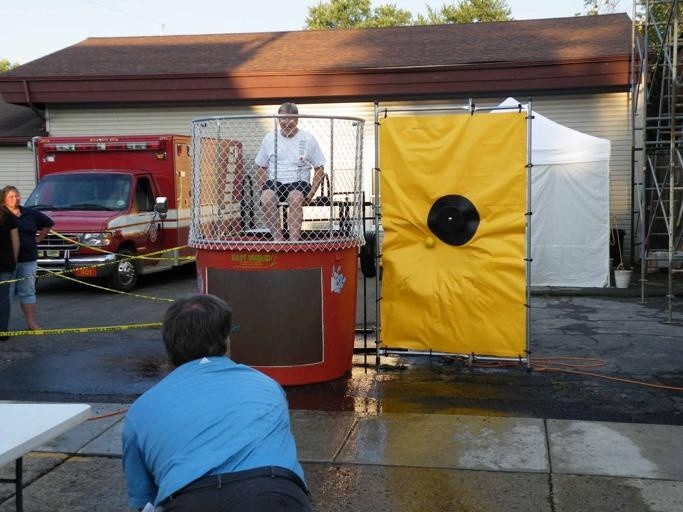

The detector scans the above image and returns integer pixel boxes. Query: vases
[613,269,631,289]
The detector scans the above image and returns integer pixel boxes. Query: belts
[159,465,312,505]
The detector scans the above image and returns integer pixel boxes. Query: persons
[3,185,55,330]
[0,189,20,340]
[122,293,313,512]
[255,102,326,242]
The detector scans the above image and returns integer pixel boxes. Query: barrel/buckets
[614,270,633,289]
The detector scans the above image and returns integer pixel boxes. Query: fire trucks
[18,130,245,292]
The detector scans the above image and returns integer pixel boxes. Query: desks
[0,397,90,512]
